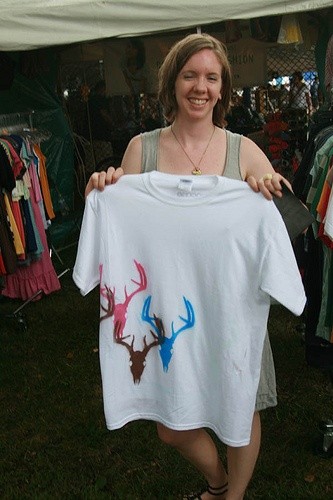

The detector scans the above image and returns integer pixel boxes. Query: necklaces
[170,124,216,177]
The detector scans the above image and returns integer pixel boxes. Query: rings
[262,174,272,180]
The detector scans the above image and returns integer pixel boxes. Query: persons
[266,71,288,116]
[118,38,149,95]
[82,32,296,500]
[87,80,117,166]
[309,77,319,111]
[290,71,312,154]
